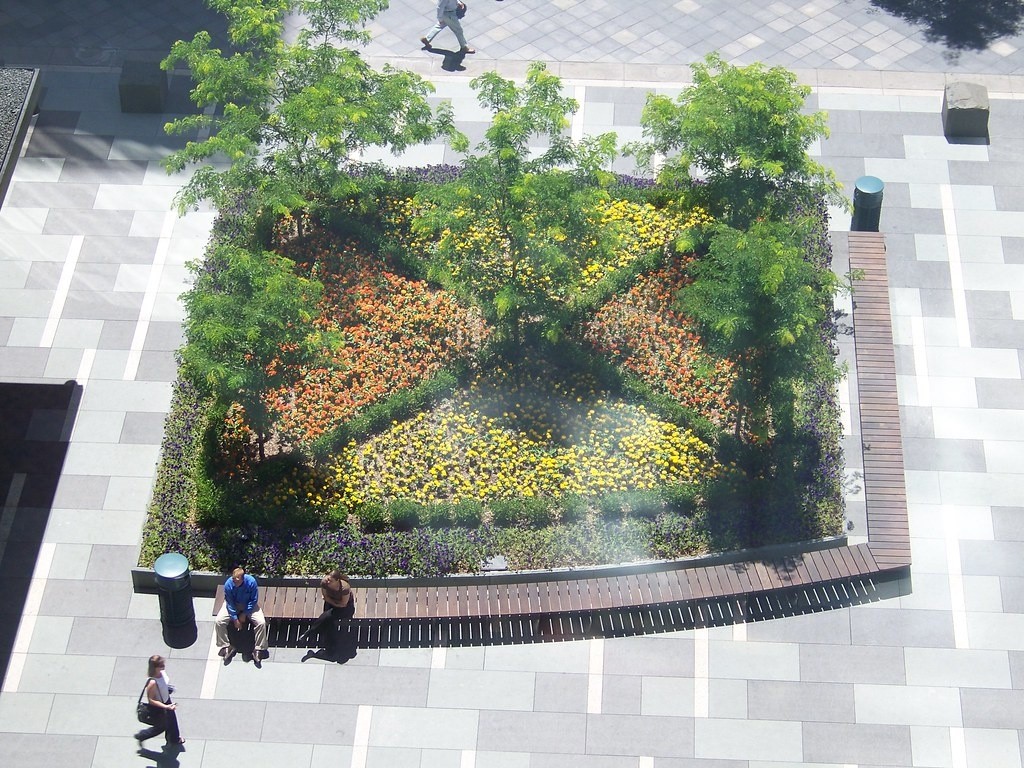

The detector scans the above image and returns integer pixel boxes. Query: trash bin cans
[850,175,885,233]
[153,554,196,631]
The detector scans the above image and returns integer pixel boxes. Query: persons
[297,570,355,657]
[214,567,269,667]
[133,654,185,745]
[420,0,475,53]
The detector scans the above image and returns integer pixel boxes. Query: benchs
[214,542,880,630]
[849,228,913,571]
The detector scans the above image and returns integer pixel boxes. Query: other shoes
[252,649,261,667]
[299,628,312,641]
[168,738,185,745]
[135,735,142,748]
[224,645,236,662]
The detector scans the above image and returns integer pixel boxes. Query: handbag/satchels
[137,678,166,726]
